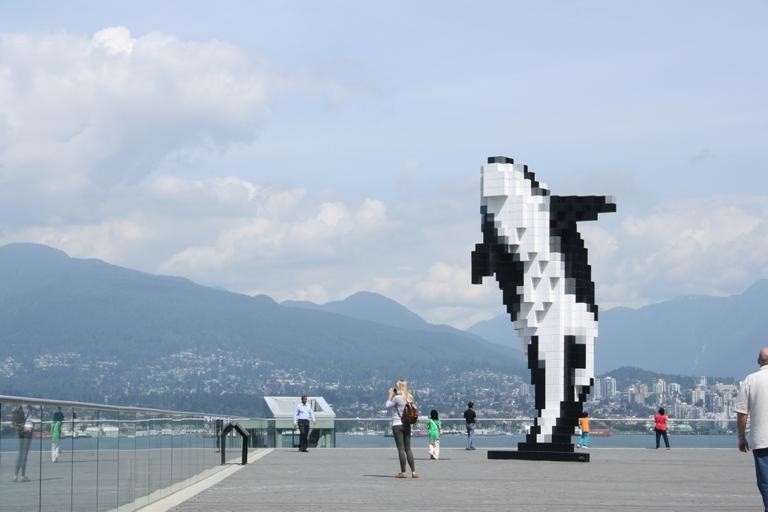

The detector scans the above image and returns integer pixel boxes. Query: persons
[51,406,64,463]
[730,346,767,510]
[464,401,476,449]
[385,379,419,478]
[293,394,316,452]
[576,410,589,448]
[654,407,670,449]
[10,403,34,481]
[425,409,441,460]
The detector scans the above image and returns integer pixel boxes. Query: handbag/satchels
[395,395,418,425]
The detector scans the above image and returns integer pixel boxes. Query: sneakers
[298,449,309,453]
[465,446,476,451]
[429,454,438,461]
[576,444,589,450]
[395,472,420,479]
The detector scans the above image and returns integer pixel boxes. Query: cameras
[393,387,397,394]
